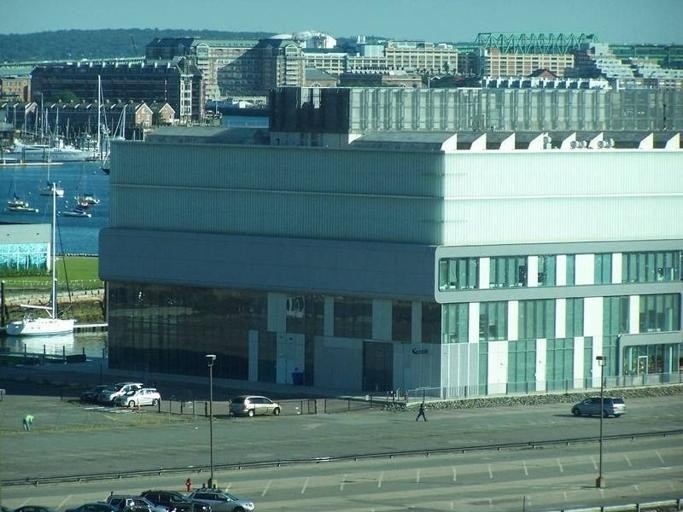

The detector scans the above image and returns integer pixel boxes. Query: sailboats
[5,181,78,338]
[6,72,131,219]
[5,332,76,362]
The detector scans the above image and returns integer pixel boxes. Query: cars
[0,484,256,512]
[228,393,282,417]
[78,381,163,410]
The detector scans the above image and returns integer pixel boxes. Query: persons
[416,404,427,422]
[23,414,34,430]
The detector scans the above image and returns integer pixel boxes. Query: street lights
[595,356,608,490]
[206,352,218,476]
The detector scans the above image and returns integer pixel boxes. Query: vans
[567,396,627,419]
